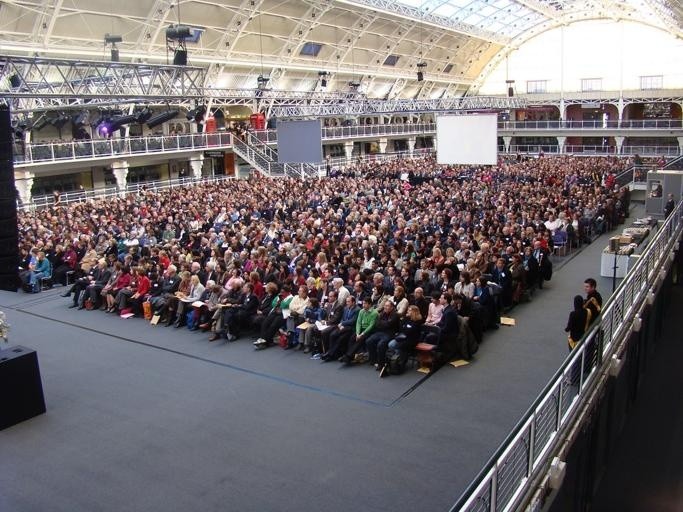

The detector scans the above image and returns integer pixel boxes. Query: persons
[10,108,677,384]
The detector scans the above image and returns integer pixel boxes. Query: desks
[599,215,658,277]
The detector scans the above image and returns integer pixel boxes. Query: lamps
[346,12,361,89]
[173,0,187,66]
[319,47,329,86]
[105,32,124,62]
[254,0,273,92]
[507,79,515,97]
[412,10,429,81]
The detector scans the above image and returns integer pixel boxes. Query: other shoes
[59,290,371,363]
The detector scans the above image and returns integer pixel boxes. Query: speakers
[173,49,187,66]
[9,75,21,87]
[508,86,513,97]
[417,72,424,81]
[321,79,326,87]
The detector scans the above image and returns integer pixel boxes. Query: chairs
[16,172,632,371]
